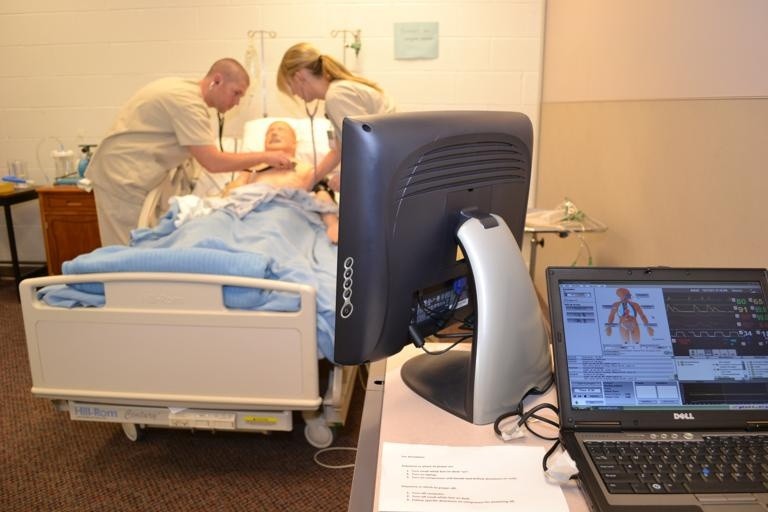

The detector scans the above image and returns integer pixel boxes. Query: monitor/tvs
[333,111,555,426]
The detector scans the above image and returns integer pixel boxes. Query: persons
[223,120,339,246]
[82,57,291,247]
[277,42,398,197]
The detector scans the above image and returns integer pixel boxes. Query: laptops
[545,266,768,512]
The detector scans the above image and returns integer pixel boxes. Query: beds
[18,112,360,448]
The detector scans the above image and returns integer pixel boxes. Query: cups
[7,160,31,189]
[53,144,75,179]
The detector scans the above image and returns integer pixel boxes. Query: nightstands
[35,182,102,278]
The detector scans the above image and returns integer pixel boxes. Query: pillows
[242,117,340,173]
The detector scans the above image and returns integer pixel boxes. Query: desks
[0,179,46,302]
[348,333,594,512]
